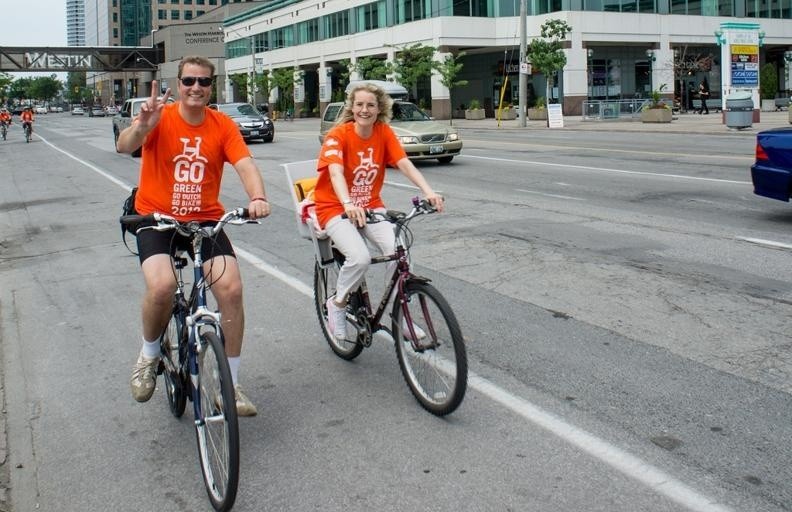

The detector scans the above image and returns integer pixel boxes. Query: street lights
[217,21,257,101]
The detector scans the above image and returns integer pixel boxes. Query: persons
[0,109,12,132]
[697,82,710,115]
[314,82,445,341]
[115,55,270,418]
[20,106,34,137]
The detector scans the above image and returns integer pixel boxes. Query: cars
[320,80,462,164]
[37,107,47,114]
[50,107,58,113]
[72,106,84,115]
[111,96,175,157]
[105,106,119,116]
[206,102,275,144]
[751,128,791,205]
[57,107,64,113]
[89,106,105,117]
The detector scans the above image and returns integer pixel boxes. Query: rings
[439,198,443,202]
[262,209,267,213]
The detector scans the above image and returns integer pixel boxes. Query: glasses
[179,76,213,88]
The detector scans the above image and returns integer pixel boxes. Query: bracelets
[339,196,353,206]
[251,196,268,203]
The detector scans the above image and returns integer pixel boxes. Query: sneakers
[325,295,347,342]
[130,348,161,403]
[401,315,427,342]
[214,384,258,418]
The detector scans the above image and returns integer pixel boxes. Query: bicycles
[122,205,263,512]
[20,120,34,144]
[1,125,9,140]
[281,158,468,417]
